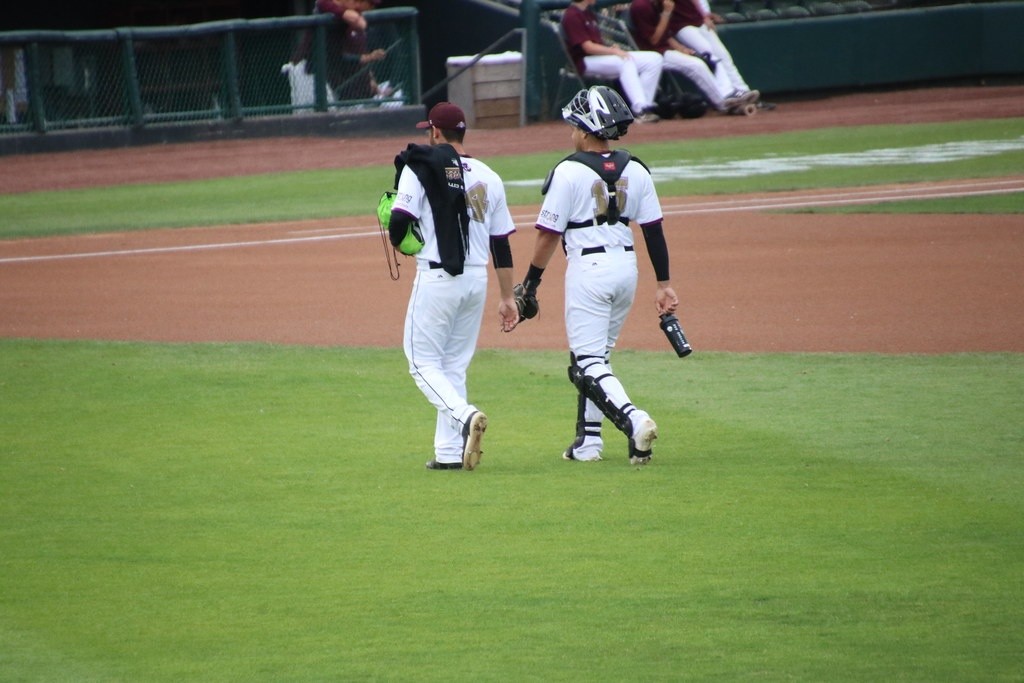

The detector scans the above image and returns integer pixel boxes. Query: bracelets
[526,263,545,279]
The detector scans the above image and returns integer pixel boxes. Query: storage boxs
[445,51,523,129]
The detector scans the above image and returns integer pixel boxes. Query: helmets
[561,85,635,141]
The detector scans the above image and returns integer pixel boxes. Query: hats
[415,102,466,129]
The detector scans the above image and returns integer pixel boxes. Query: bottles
[658,314,692,358]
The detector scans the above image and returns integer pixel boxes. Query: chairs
[538,0,889,122]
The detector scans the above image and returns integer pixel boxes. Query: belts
[429,262,444,269]
[581,245,634,255]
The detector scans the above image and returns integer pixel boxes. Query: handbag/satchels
[700,51,717,74]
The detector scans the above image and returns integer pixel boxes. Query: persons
[282,0,403,116]
[630,0,762,115]
[387,103,520,471]
[512,86,678,463]
[561,0,663,122]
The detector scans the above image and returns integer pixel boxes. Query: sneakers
[426,460,462,469]
[724,88,760,108]
[563,435,603,461]
[628,409,657,465]
[462,411,488,471]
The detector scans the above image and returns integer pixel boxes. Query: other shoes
[756,101,776,111]
[634,110,660,122]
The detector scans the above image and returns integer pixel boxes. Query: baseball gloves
[499,278,542,333]
